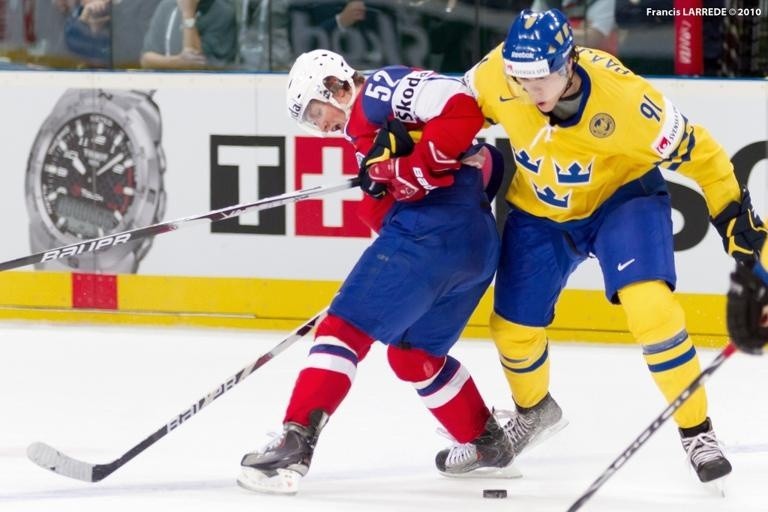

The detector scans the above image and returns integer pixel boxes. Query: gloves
[358,117,459,201]
[726,271,768,355]
[709,183,768,268]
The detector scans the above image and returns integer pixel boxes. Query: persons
[359,9,768,483]
[241,49,515,477]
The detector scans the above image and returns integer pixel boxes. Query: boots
[240,409,330,477]
[435,391,563,473]
[677,418,733,482]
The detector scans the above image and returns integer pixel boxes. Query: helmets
[285,48,356,124]
[502,1,578,79]
